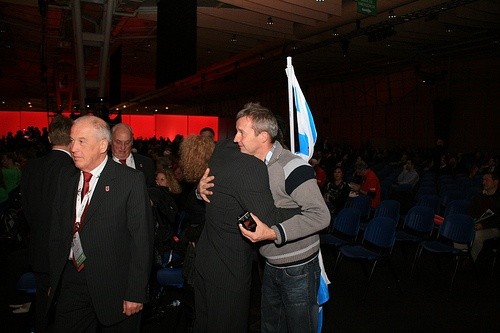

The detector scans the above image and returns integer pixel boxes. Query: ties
[71,171,93,272]
[119,159,126,164]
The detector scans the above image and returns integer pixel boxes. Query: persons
[195,103,331,333]
[194,140,304,333]
[0,126,218,307]
[110,122,155,188]
[309,144,381,220]
[428,139,500,179]
[394,155,419,185]
[450,173,499,263]
[47,115,153,333]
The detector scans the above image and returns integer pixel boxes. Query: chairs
[154,201,205,302]
[320,156,487,291]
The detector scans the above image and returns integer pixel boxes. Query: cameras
[237,210,257,232]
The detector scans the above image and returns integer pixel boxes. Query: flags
[285,65,331,332]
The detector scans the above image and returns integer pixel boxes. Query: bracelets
[196,190,202,200]
